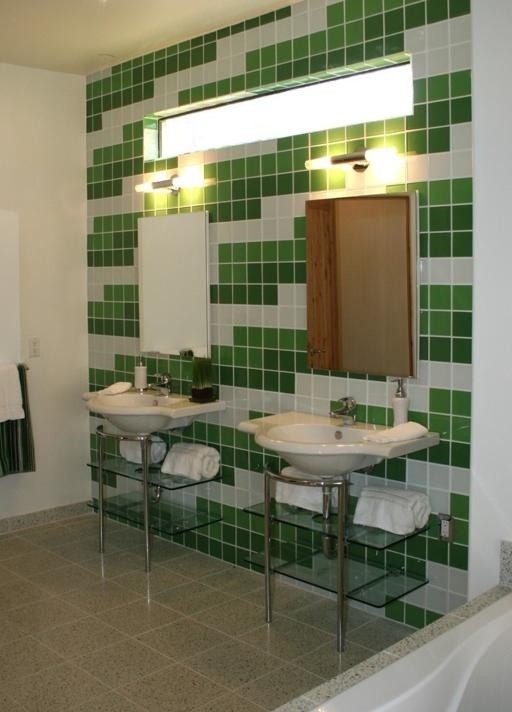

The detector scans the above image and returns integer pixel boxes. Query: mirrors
[304,191,420,377]
[137,210,214,362]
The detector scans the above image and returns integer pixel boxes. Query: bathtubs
[309,588,512,708]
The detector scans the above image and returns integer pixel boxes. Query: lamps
[305,146,400,180]
[136,169,203,194]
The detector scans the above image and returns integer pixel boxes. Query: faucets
[326,396,356,425]
[136,372,170,397]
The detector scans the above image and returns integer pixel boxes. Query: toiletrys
[134,356,148,391]
[392,379,409,427]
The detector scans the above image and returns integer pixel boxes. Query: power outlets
[439,520,454,544]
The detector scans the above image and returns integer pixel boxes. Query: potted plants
[191,357,214,404]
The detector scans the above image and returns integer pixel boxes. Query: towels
[118,435,164,465]
[161,441,223,483]
[367,421,432,442]
[0,364,25,422]
[101,381,133,394]
[0,365,35,477]
[274,465,338,514]
[351,483,432,535]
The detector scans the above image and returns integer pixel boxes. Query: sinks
[234,409,439,479]
[79,388,226,438]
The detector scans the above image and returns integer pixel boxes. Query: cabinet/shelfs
[241,500,431,650]
[86,458,224,572]
